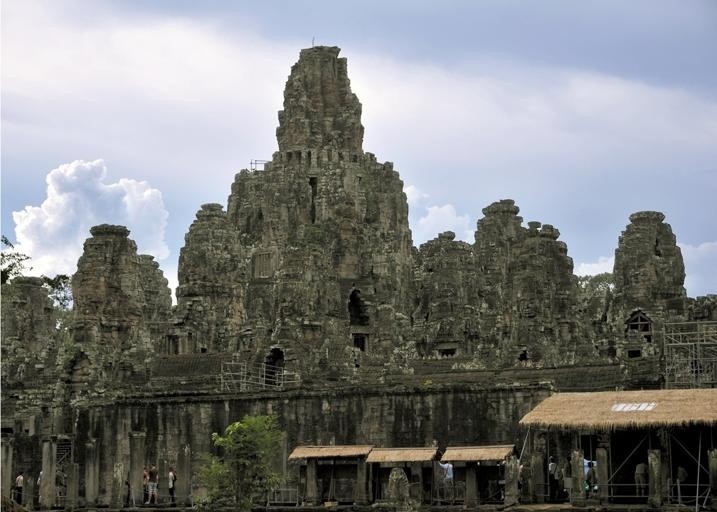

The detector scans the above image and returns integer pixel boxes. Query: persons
[16,461,178,509]
[438,459,455,494]
[517,453,688,499]
